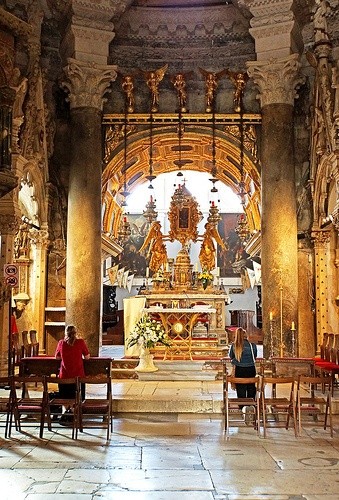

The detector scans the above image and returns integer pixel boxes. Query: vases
[134,349,159,372]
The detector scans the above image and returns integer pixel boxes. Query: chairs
[222,373,334,438]
[309,333,339,394]
[0,375,114,440]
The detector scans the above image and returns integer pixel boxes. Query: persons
[54,325,90,426]
[228,326,258,425]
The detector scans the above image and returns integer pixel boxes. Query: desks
[18,356,112,421]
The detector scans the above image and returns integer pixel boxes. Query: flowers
[125,311,173,349]
[199,272,214,290]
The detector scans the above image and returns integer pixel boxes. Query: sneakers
[241,405,250,426]
[248,406,256,425]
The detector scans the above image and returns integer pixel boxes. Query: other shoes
[59,410,74,426]
